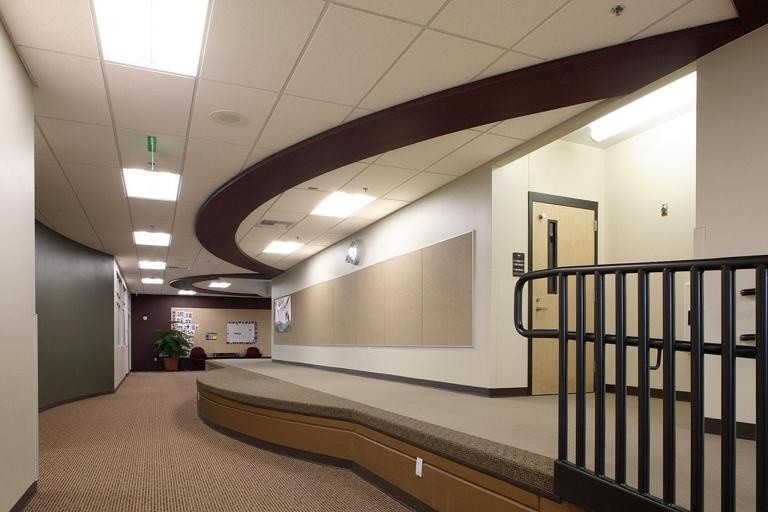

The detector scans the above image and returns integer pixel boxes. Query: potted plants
[152,329,190,371]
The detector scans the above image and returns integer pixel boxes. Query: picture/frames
[207,333,217,340]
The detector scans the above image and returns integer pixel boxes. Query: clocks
[346,240,360,266]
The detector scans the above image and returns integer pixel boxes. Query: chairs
[189,346,207,370]
[245,347,262,358]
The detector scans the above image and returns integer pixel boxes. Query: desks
[213,353,239,359]
